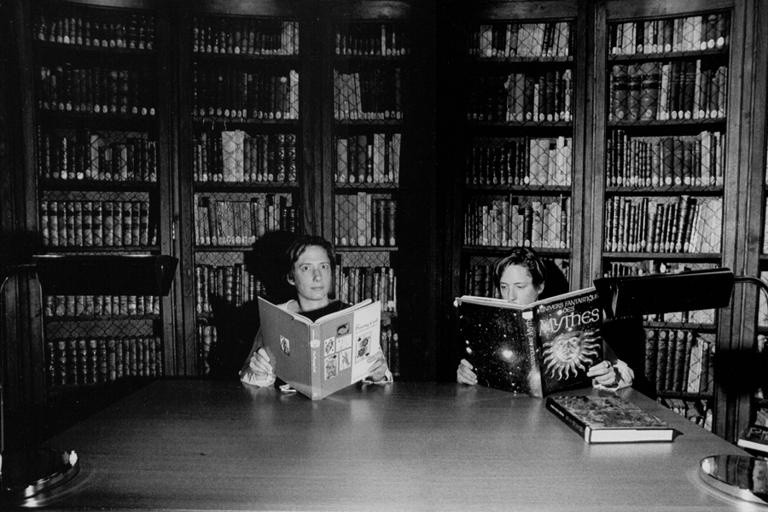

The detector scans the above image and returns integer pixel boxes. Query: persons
[455,247,635,393]
[243,238,393,387]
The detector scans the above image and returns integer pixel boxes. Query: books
[196,264,266,375]
[257,296,382,401]
[739,271,768,450]
[39,252,163,384]
[193,15,299,249]
[333,22,403,246]
[600,12,721,255]
[457,286,603,400]
[603,262,713,435]
[335,253,399,380]
[465,24,574,247]
[464,256,569,299]
[546,397,674,443]
[33,5,159,247]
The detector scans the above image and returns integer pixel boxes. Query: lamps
[0,252,179,509]
[593,267,768,493]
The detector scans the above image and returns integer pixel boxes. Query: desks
[0,375,768,512]
